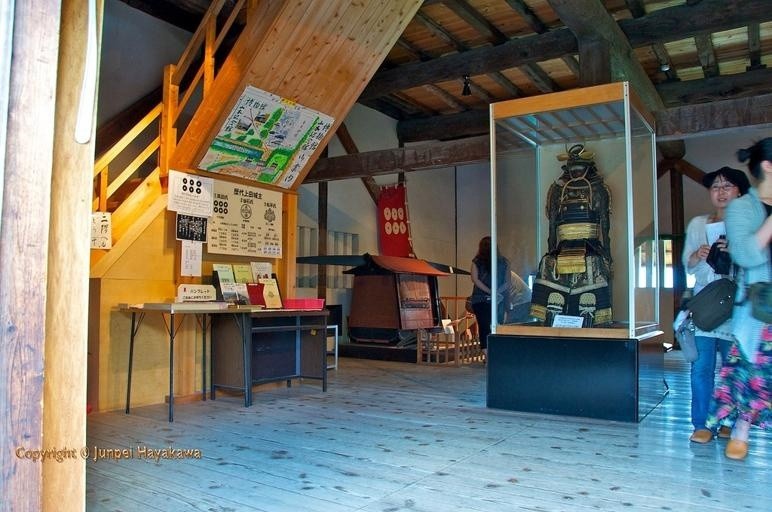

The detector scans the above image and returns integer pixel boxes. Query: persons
[725,136,772,460]
[681,165,753,444]
[471,236,511,366]
[505,270,532,325]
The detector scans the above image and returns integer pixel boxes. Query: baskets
[284,298,325,309]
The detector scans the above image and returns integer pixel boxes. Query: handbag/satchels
[685,277,737,332]
[675,326,699,362]
[749,282,772,323]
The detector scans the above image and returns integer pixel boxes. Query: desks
[113,299,264,422]
[208,306,331,407]
[326,323,340,371]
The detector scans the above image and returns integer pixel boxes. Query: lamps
[459,71,475,99]
[655,60,674,76]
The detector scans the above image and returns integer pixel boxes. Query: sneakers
[690,430,712,443]
[726,438,748,459]
[719,426,731,438]
